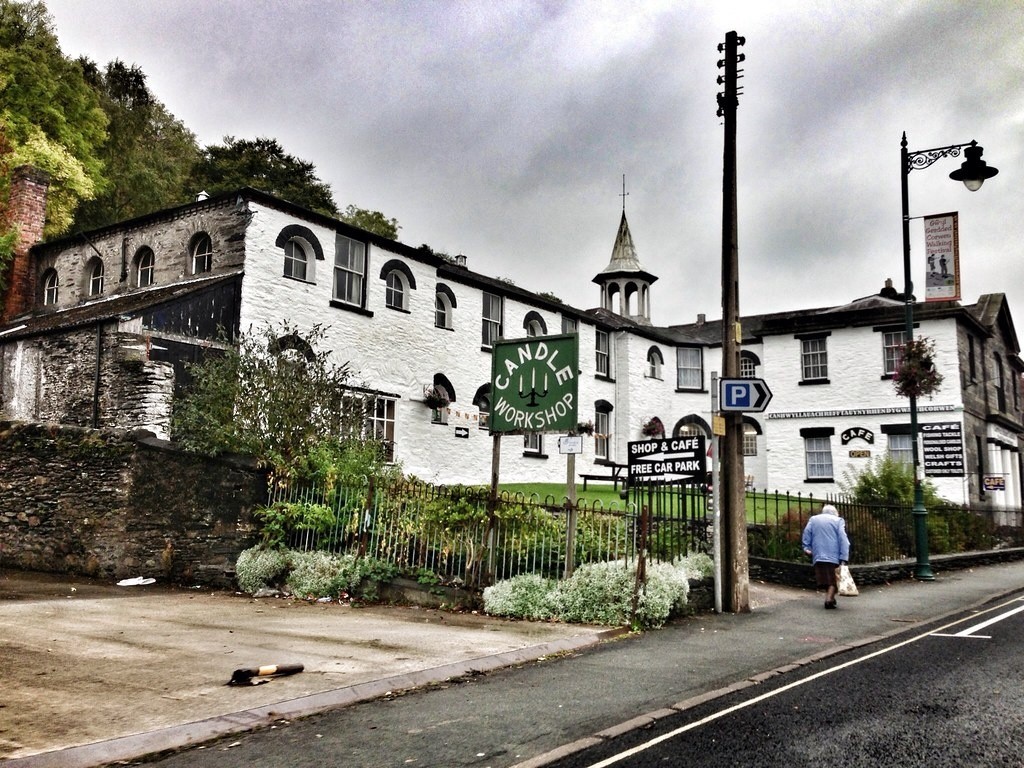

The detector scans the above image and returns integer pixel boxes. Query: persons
[801,504,851,610]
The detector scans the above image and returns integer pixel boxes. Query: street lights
[898,131,999,580]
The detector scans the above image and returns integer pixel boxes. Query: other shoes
[824,595,837,610]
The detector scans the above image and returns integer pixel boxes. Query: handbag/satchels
[835,561,859,596]
